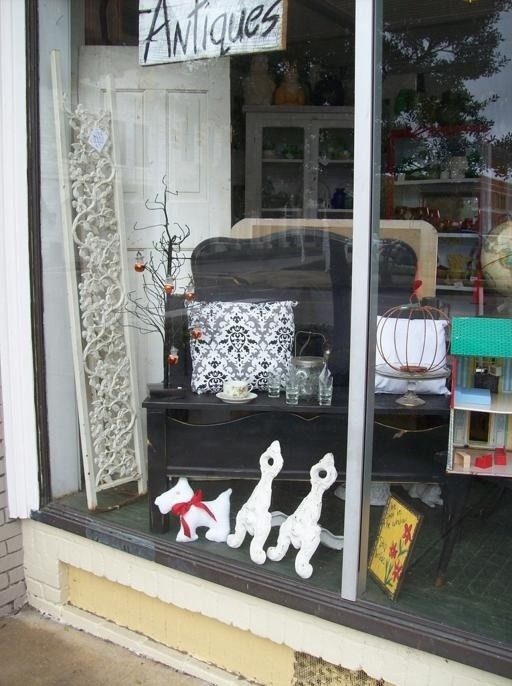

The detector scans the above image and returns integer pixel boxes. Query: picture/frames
[367,493,423,604]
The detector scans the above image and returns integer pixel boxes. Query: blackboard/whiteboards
[135,1,287,67]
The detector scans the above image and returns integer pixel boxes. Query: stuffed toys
[154,478,233,543]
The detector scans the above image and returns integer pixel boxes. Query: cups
[318,376,333,397]
[267,370,281,397]
[222,380,253,397]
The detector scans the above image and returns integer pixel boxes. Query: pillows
[182,299,298,394]
[375,314,451,395]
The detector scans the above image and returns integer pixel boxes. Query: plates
[216,393,258,405]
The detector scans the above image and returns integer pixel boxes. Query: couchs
[164,229,440,381]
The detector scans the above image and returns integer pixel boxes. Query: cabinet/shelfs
[142,390,465,545]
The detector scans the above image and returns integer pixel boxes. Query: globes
[478,214,512,318]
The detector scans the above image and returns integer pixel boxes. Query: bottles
[285,385,299,405]
[291,354,327,399]
